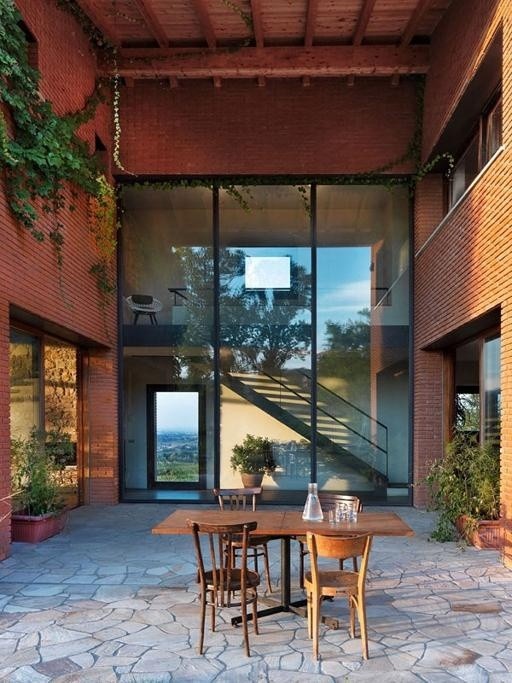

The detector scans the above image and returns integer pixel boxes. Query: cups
[328,502,357,525]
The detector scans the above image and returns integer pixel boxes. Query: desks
[152,509,415,629]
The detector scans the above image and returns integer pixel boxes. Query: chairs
[126,295,163,326]
[197,488,371,665]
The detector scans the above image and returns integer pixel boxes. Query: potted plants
[230,436,278,492]
[13,427,75,544]
[431,442,508,548]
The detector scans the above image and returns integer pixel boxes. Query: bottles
[300,482,325,522]
